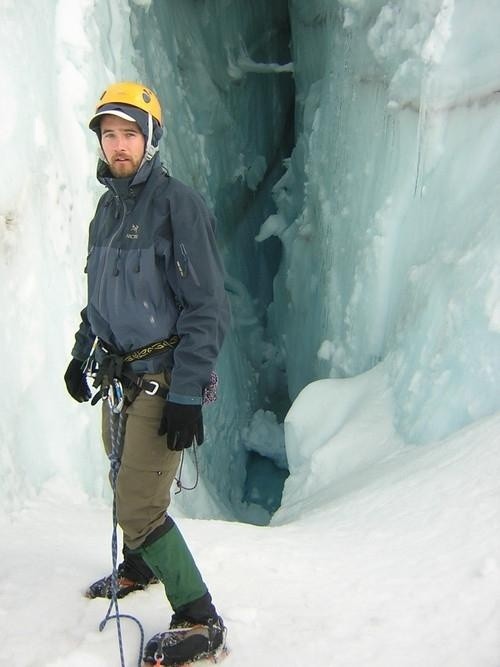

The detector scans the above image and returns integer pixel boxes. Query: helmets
[95,82,162,147]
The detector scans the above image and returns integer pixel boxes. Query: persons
[63,81,232,667]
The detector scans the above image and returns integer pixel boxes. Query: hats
[88,111,137,129]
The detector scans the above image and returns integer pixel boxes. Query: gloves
[157,401,204,451]
[64,359,91,402]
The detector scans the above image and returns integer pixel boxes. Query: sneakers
[87,566,160,598]
[146,619,222,667]
